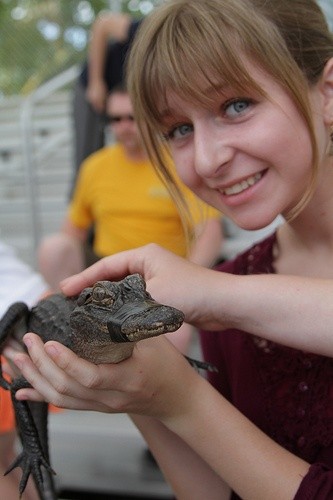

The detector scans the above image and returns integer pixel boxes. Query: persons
[37,13,223,354]
[0,0,333,500]
[0,238,62,500]
[59,242,333,357]
